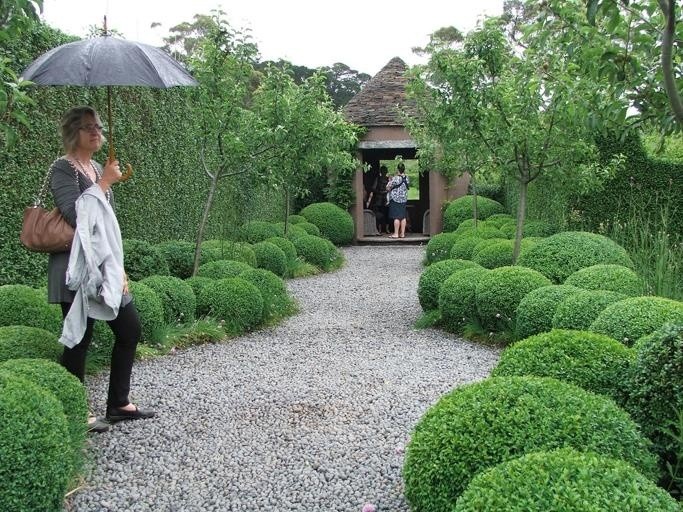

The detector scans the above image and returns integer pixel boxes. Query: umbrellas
[17,16,201,182]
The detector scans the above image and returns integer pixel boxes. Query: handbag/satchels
[21,204,75,252]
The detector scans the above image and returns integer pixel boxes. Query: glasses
[79,124,101,131]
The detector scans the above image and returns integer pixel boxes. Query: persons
[48,106,156,432]
[366,163,409,238]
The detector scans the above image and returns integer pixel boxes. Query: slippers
[389,234,405,238]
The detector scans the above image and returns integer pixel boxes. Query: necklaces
[72,152,110,204]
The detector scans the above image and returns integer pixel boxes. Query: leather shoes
[91,407,155,435]
